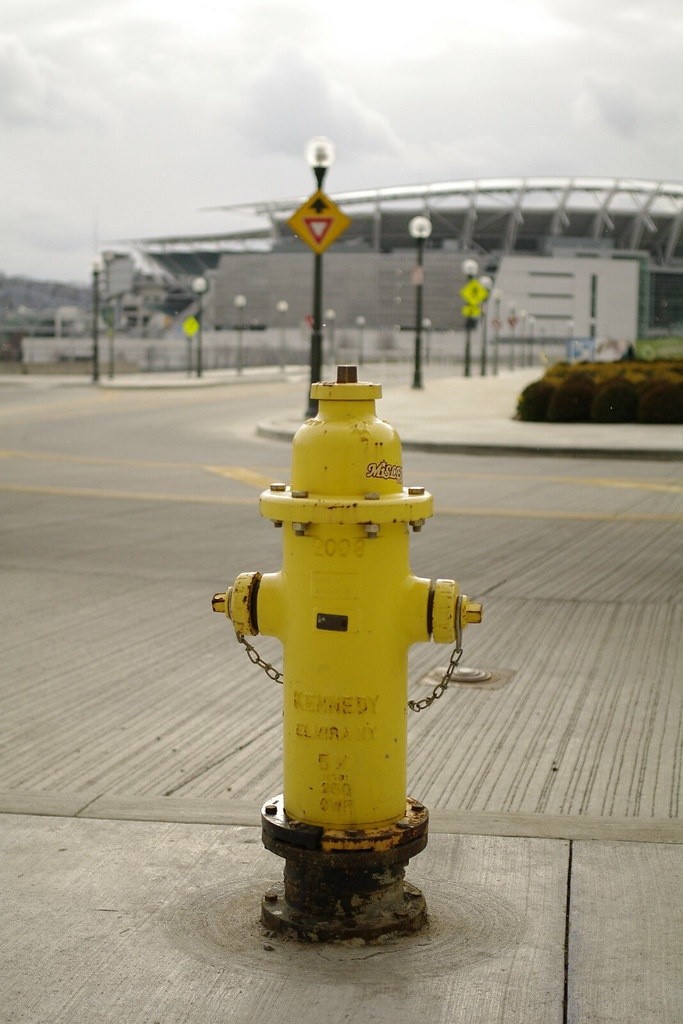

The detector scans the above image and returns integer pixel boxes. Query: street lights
[234,293,247,378]
[407,215,434,389]
[304,138,334,414]
[461,258,480,379]
[481,275,535,377]
[191,275,208,380]
[276,300,291,378]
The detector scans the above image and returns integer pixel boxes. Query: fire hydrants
[209,365,482,941]
